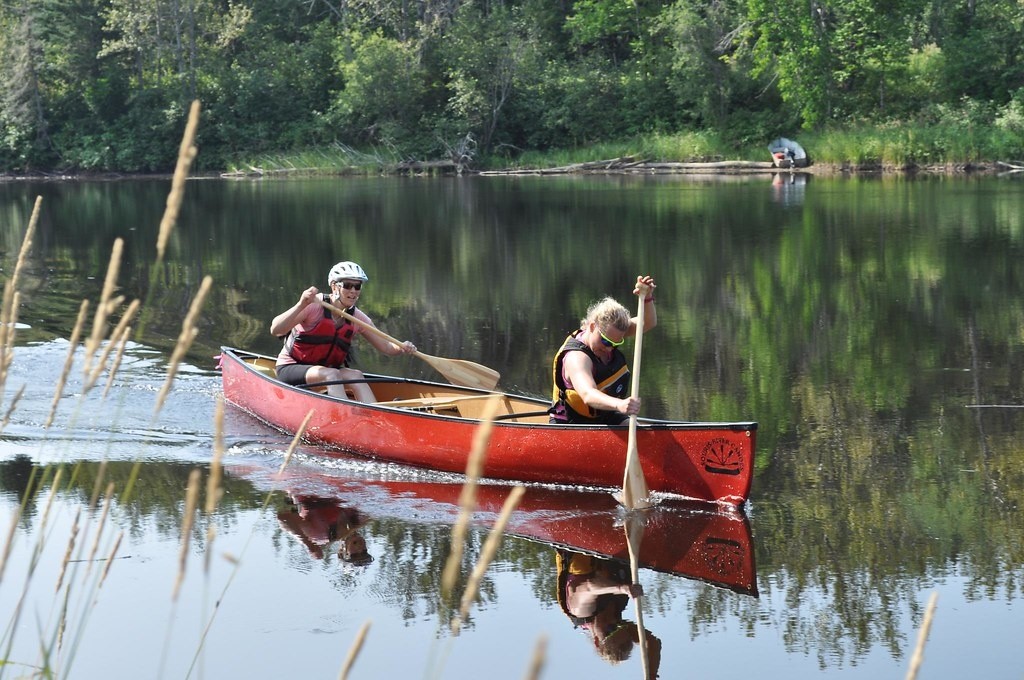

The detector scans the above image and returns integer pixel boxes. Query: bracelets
[645,296,655,304]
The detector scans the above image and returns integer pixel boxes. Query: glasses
[334,281,361,291]
[593,321,624,348]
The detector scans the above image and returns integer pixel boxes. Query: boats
[215,343,758,512]
[212,409,759,601]
[767,135,809,168]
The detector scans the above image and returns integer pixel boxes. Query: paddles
[623,503,650,679]
[621,282,650,513]
[309,293,500,392]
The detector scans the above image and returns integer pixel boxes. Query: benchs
[374,395,507,407]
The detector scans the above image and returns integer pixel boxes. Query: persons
[546,276,658,425]
[278,488,375,567]
[269,260,418,403]
[555,544,662,680]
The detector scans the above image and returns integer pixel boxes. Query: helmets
[328,261,368,286]
